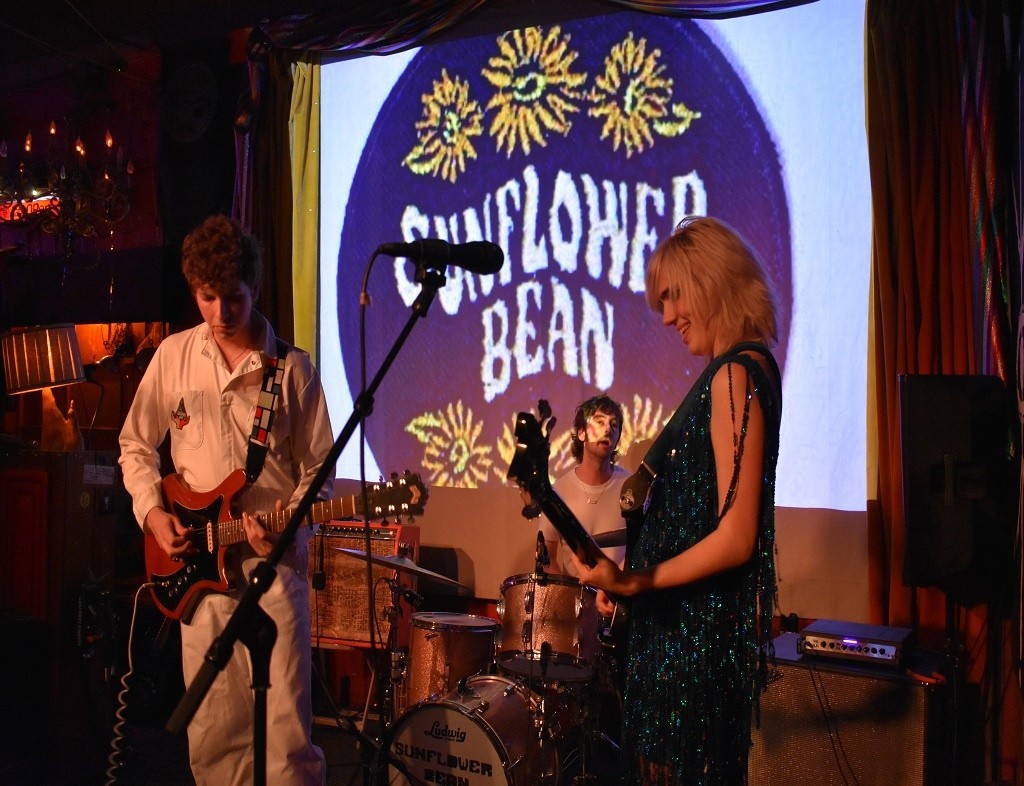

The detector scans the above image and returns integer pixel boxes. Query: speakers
[897,373,1022,590]
[748,633,980,786]
[305,519,420,649]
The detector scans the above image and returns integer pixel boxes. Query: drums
[404,612,500,715]
[380,673,560,786]
[498,572,605,683]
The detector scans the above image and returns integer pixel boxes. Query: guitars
[503,396,626,690]
[141,466,429,624]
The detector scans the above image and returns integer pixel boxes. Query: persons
[539,394,647,582]
[564,216,783,786]
[118,213,336,786]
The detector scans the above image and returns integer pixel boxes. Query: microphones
[312,541,326,590]
[380,240,504,275]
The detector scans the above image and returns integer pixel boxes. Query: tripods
[562,707,623,786]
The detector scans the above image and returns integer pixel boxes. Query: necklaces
[577,470,611,504]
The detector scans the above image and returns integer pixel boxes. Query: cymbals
[329,545,471,590]
[592,527,627,548]
